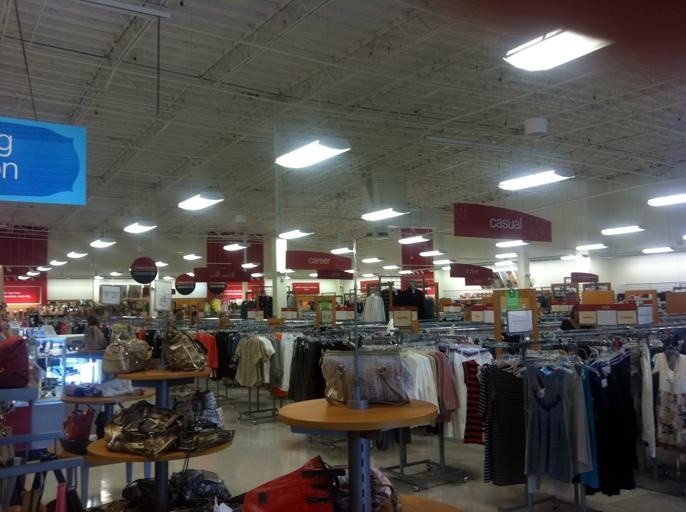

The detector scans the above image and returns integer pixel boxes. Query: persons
[561,306,580,330]
[83,317,109,349]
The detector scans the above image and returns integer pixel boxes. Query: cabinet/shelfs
[84,361,233,511]
[278,399,458,512]
[27,336,105,450]
[47,387,155,464]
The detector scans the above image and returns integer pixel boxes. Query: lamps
[363,204,410,222]
[19,239,119,287]
[278,226,315,240]
[497,166,575,190]
[274,137,352,171]
[178,191,225,212]
[502,27,615,72]
[122,221,160,235]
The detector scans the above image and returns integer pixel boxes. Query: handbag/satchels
[318,348,411,406]
[242,455,402,512]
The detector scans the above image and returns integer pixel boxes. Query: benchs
[1,388,86,512]
